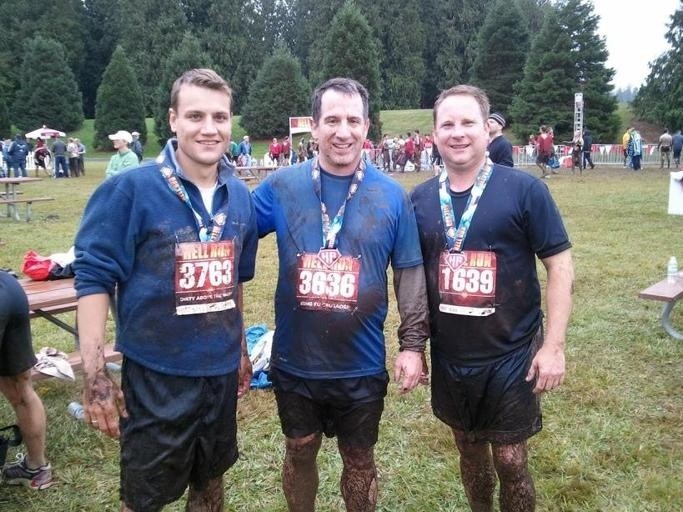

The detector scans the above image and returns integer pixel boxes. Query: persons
[536,125,553,178]
[398,135,404,146]
[1,270,54,490]
[412,138,416,148]
[33,144,53,177]
[423,134,433,148]
[238,135,252,166]
[623,127,631,167]
[362,137,382,169]
[67,138,80,178]
[251,78,428,512]
[399,132,421,173]
[657,128,672,169]
[52,134,68,178]
[36,137,42,146]
[583,129,595,170]
[631,130,643,170]
[128,132,143,164]
[105,130,138,177]
[488,111,514,167]
[225,140,241,175]
[75,69,259,512]
[672,130,683,167]
[529,134,538,144]
[410,85,574,511]
[545,127,560,174]
[74,138,86,177]
[10,134,29,177]
[282,136,293,166]
[268,138,282,167]
[412,130,424,174]
[563,131,584,177]
[1,137,10,177]
[298,137,318,162]
[392,137,400,172]
[291,149,298,164]
[430,142,444,169]
[382,134,393,172]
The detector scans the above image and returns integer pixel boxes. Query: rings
[89,418,97,422]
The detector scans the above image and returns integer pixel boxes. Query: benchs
[0,197,56,223]
[30,344,121,382]
[638,269,683,340]
[237,176,266,183]
[0,189,24,215]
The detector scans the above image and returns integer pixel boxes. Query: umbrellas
[24,123,68,145]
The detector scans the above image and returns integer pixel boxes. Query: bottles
[666,255,678,287]
[105,362,121,370]
[68,401,84,420]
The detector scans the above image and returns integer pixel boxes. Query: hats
[108,130,133,144]
[131,131,140,136]
[489,112,506,128]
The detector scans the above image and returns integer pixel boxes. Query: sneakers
[2,454,54,491]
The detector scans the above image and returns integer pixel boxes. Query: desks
[235,166,282,183]
[0,176,43,221]
[0,276,122,381]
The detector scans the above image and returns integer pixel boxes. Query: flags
[288,116,313,164]
